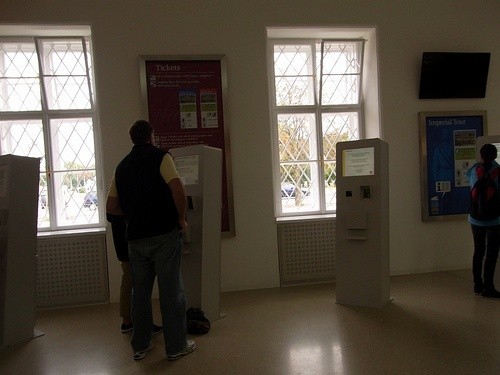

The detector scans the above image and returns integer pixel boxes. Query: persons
[106,213,162,335]
[461,144,500,299]
[106,121,197,360]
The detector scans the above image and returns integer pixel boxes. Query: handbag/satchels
[187,307,211,335]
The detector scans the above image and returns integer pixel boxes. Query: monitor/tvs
[0,164,8,194]
[418,51,491,99]
[341,146,375,176]
[490,142,500,166]
[172,154,198,186]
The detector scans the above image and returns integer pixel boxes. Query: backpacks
[469,165,500,221]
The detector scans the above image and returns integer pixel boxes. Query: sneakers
[167,340,196,360]
[133,341,154,359]
[153,324,162,334]
[120,323,134,332]
[482,290,500,298]
[474,286,481,293]
